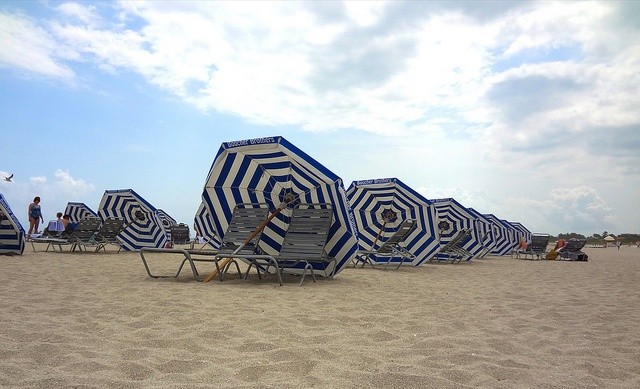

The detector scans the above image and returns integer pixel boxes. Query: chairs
[351,217,419,273]
[54,207,124,257]
[30,207,102,253]
[543,235,588,263]
[214,202,339,286]
[510,233,552,262]
[141,201,270,280]
[429,227,476,267]
[170,223,191,249]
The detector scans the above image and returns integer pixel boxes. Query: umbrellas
[345,179,441,272]
[201,136,359,285]
[158,208,176,241]
[467,208,497,260]
[98,190,167,255]
[511,221,530,252]
[195,202,222,250]
[0,193,26,255]
[501,220,519,253]
[428,198,484,265]
[64,202,97,229]
[485,213,509,256]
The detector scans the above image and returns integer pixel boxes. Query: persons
[28,197,44,241]
[39,211,70,237]
